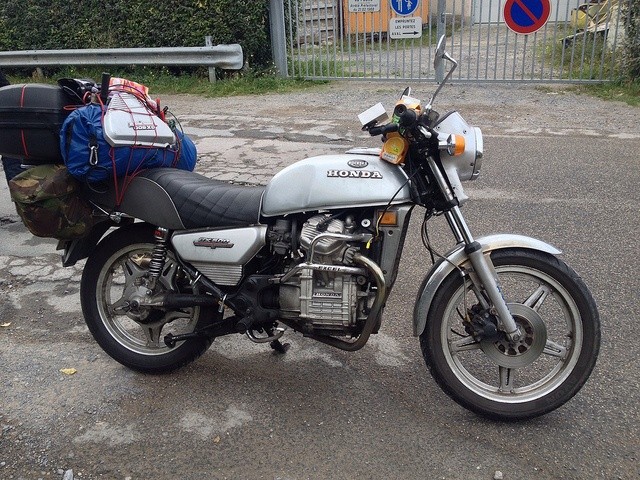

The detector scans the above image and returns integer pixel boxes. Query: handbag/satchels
[59,102,197,180]
[10,166,111,238]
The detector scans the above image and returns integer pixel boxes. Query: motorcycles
[1,35,602,423]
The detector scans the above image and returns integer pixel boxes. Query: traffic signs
[390,17,423,39]
